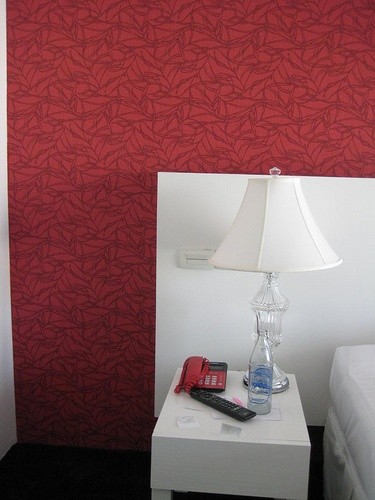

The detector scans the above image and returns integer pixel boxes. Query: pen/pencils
[233,398,246,408]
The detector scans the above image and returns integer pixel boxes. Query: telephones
[182,355,227,390]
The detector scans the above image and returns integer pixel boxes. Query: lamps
[209,167,343,394]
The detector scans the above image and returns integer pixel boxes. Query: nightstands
[150,367,311,500]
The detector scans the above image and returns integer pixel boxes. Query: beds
[323,345,375,500]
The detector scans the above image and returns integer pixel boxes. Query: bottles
[247,330,273,416]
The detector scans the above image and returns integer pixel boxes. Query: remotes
[190,389,255,422]
